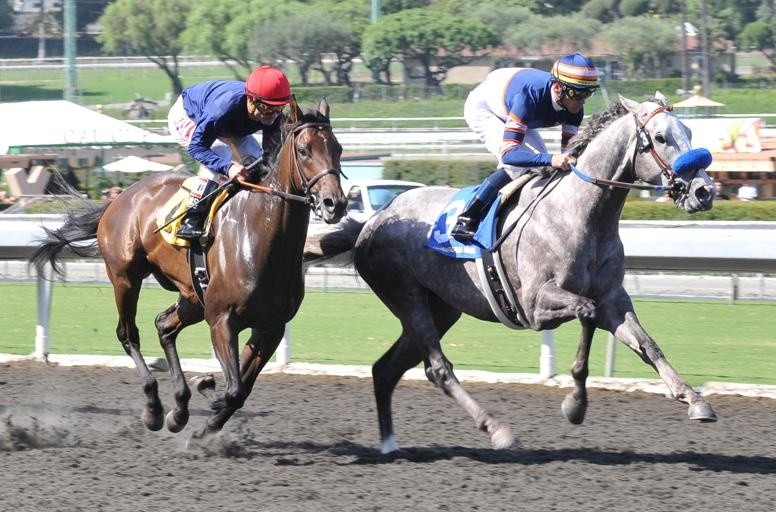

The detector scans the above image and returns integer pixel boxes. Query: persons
[109,182,123,198]
[156,65,294,239]
[714,177,759,201]
[451,53,601,238]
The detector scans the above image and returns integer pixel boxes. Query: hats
[548,53,599,88]
[244,64,294,106]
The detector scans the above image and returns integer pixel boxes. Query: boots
[177,178,224,239]
[450,178,500,241]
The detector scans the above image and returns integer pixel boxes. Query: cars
[340,180,428,223]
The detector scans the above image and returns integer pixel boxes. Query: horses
[304,88,718,458]
[26,91,350,443]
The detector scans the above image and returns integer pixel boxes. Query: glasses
[254,102,286,115]
[566,87,593,100]
